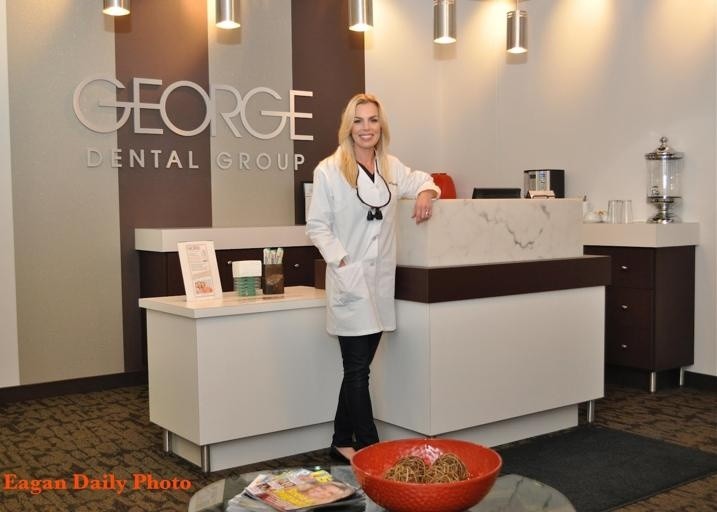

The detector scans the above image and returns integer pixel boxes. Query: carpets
[480,425,717,512]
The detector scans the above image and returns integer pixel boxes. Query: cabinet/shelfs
[140,245,316,376]
[584,222,697,393]
[140,284,347,473]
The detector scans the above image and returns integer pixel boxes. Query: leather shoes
[331,448,357,465]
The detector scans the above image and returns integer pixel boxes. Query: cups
[608,200,623,224]
[620,200,632,224]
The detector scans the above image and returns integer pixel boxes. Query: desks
[187,465,576,512]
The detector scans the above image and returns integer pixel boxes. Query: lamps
[348,0,373,33]
[102,0,131,17]
[507,0,528,54]
[434,0,457,45]
[215,0,241,31]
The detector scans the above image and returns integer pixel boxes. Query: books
[227,471,366,512]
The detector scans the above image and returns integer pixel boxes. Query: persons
[305,92,441,466]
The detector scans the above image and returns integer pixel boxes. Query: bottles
[647,135,686,224]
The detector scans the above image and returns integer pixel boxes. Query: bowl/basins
[352,436,503,511]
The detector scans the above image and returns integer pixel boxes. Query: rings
[426,212,428,215]
